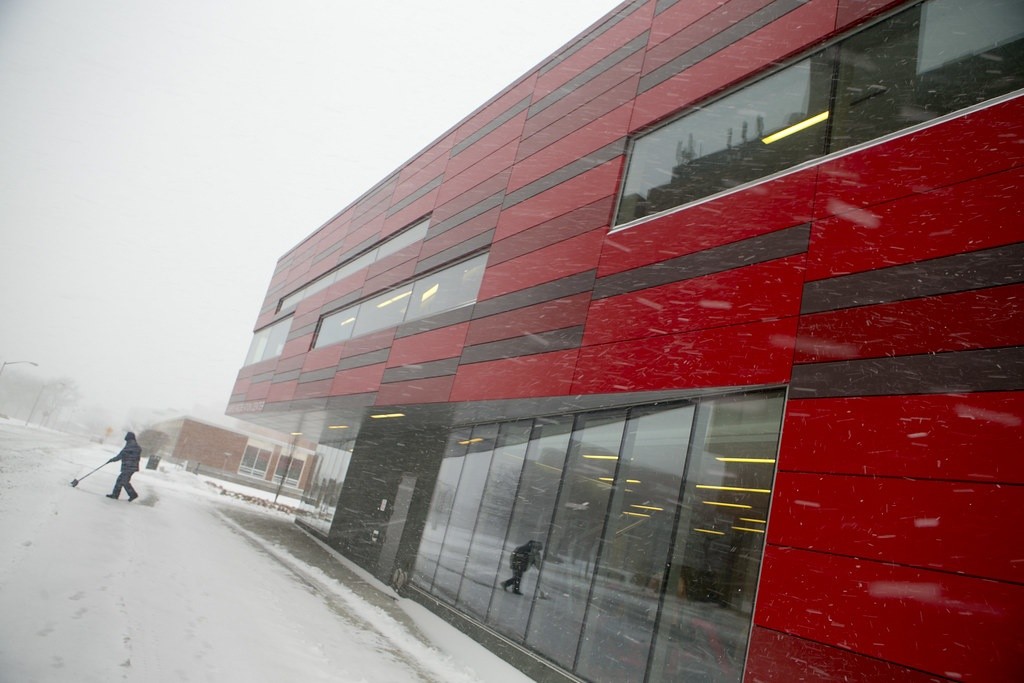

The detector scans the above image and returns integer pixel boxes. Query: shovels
[70,460,113,488]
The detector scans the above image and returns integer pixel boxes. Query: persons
[106,431,142,502]
[501,540,543,594]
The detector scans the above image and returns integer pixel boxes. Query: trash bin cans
[145,453,161,470]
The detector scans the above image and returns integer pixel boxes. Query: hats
[125,432,135,440]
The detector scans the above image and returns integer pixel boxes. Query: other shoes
[501,582,507,591]
[128,493,137,501]
[513,590,522,595]
[107,494,119,499]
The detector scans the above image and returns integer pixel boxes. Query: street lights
[0,360,39,371]
